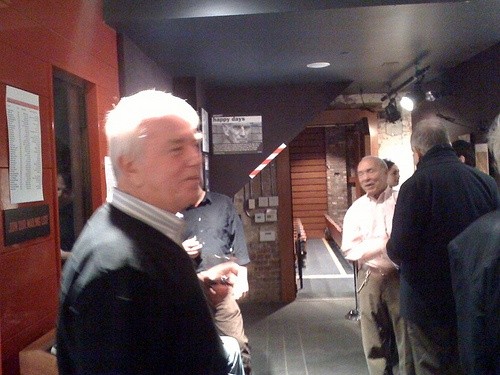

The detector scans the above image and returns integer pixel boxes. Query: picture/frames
[211,113,264,155]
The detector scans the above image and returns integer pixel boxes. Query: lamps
[380,64,436,124]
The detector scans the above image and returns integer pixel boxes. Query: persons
[56,87,252,375]
[339,156,416,375]
[218,334,246,375]
[382,159,401,190]
[450,139,476,168]
[450,113,500,375]
[382,115,498,375]
[57,164,75,262]
[177,183,252,375]
